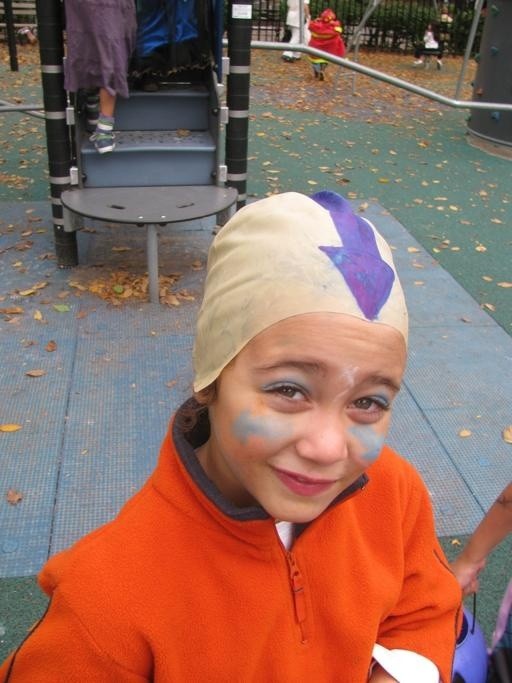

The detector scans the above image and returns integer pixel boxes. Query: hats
[193,191,409,393]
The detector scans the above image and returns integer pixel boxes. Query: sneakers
[85,97,116,156]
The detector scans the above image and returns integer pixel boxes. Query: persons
[281,0,311,63]
[448,475,512,680]
[62,0,141,158]
[413,17,439,66]
[306,7,346,83]
[129,0,213,94]
[1,188,463,682]
